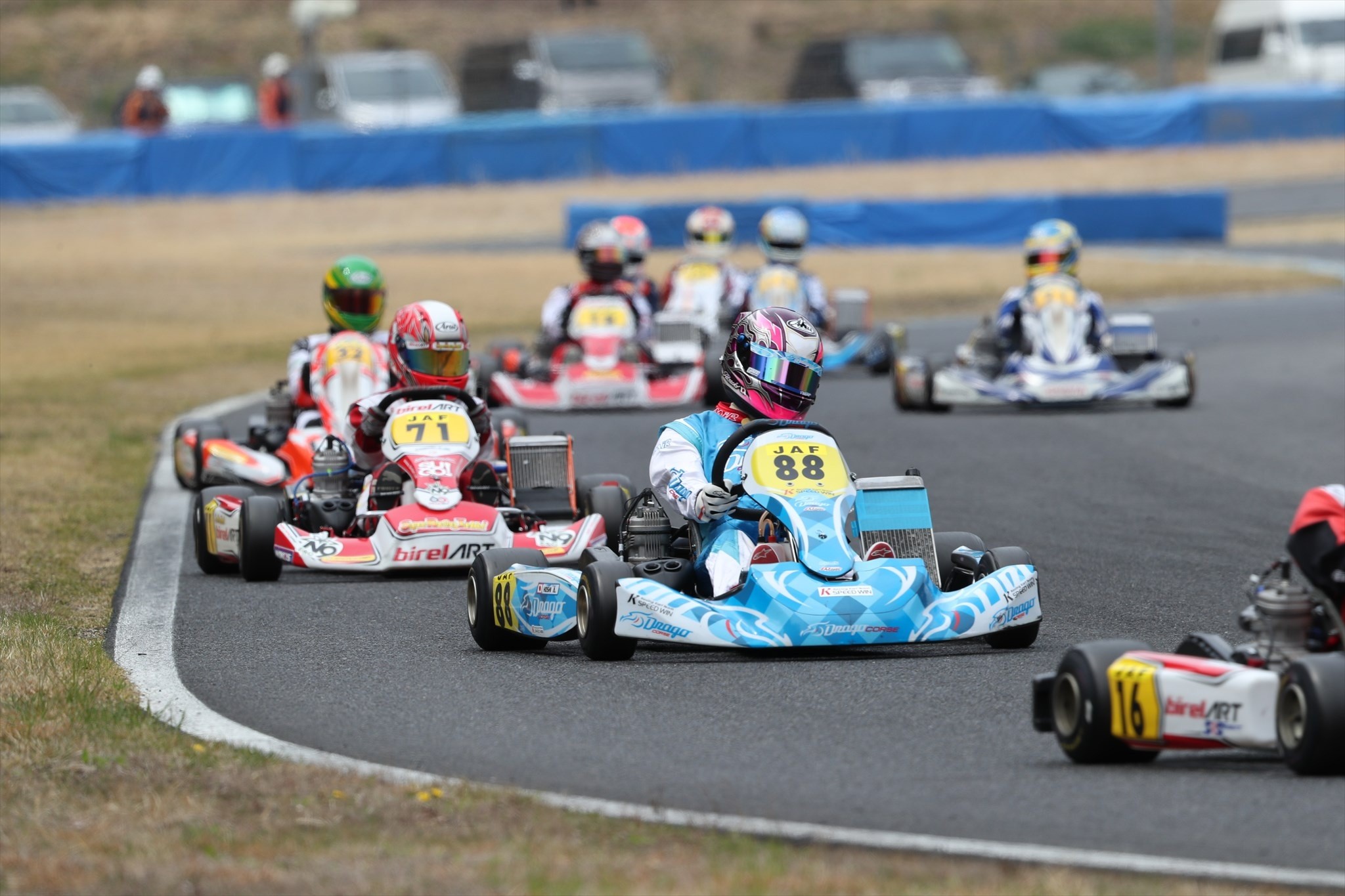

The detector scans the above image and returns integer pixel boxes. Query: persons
[258,50,295,126]
[723,204,841,343]
[287,254,397,433]
[520,207,751,383]
[341,298,547,535]
[984,215,1124,379]
[649,303,896,601]
[1287,482,1345,651]
[125,65,169,131]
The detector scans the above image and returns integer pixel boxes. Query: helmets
[608,215,652,273]
[682,205,735,259]
[323,257,385,332]
[720,307,825,421]
[1021,219,1083,282]
[388,301,470,400]
[756,207,811,263]
[577,218,628,281]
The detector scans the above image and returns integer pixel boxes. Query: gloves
[465,397,491,431]
[701,480,738,519]
[360,405,388,439]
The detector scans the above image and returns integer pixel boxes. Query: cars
[0,84,85,142]
[1015,58,1144,96]
[107,73,257,125]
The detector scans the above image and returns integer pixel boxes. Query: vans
[457,27,656,114]
[1203,0,1345,87]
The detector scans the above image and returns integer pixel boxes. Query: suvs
[285,49,457,126]
[785,29,1001,103]
